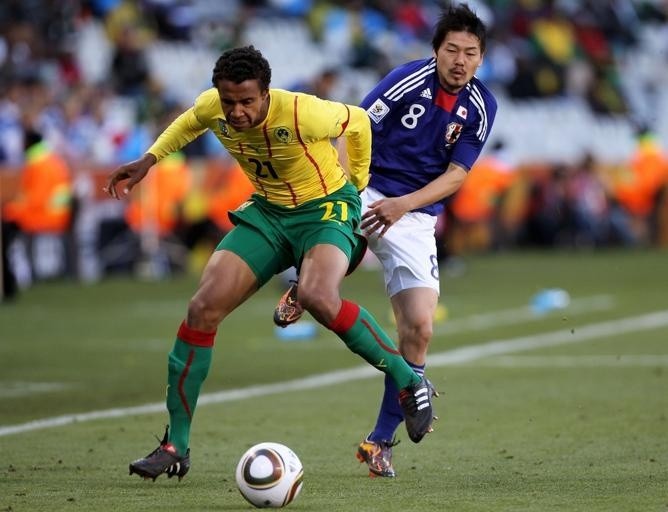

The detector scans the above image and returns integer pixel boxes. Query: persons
[98,44,443,482]
[1,1,668,305]
[273,3,502,482]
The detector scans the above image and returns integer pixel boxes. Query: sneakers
[128,442,192,482]
[355,431,397,479]
[272,279,306,328]
[399,376,439,444]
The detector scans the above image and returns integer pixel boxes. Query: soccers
[235,442,304,507]
